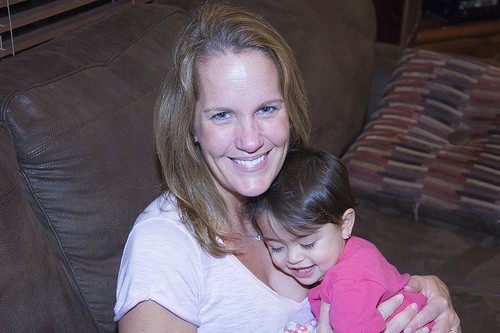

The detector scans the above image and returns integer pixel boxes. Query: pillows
[342,48,500,234]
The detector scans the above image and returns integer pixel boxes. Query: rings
[449,330,457,333]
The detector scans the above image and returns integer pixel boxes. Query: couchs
[0,0,500,333]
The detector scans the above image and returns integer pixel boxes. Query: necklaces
[229,217,261,242]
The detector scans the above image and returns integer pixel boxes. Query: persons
[246,147,437,333]
[113,2,463,333]
